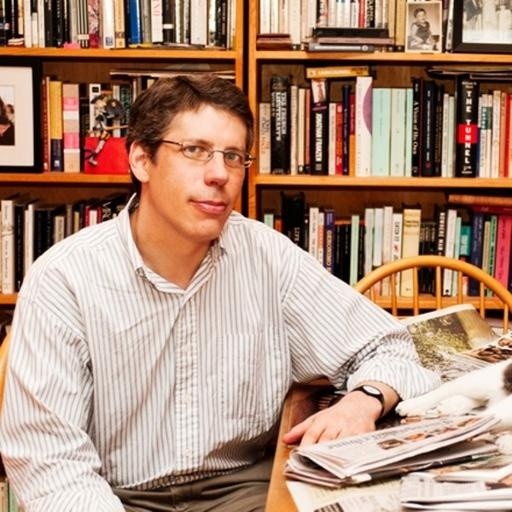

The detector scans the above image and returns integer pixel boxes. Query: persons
[2,67,444,511]
[409,7,437,50]
[496,4,510,43]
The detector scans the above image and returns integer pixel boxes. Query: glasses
[160,140,256,168]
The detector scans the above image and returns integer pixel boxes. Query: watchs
[351,384,389,414]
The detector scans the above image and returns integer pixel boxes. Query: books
[1,1,237,51]
[259,64,511,178]
[264,190,510,297]
[36,65,236,175]
[2,193,129,295]
[257,0,407,52]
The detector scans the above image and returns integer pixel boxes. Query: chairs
[346,254,510,341]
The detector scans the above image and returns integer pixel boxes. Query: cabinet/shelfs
[249,0,512,310]
[0,0,249,311]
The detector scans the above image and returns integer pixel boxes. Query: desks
[263,372,511,512]
